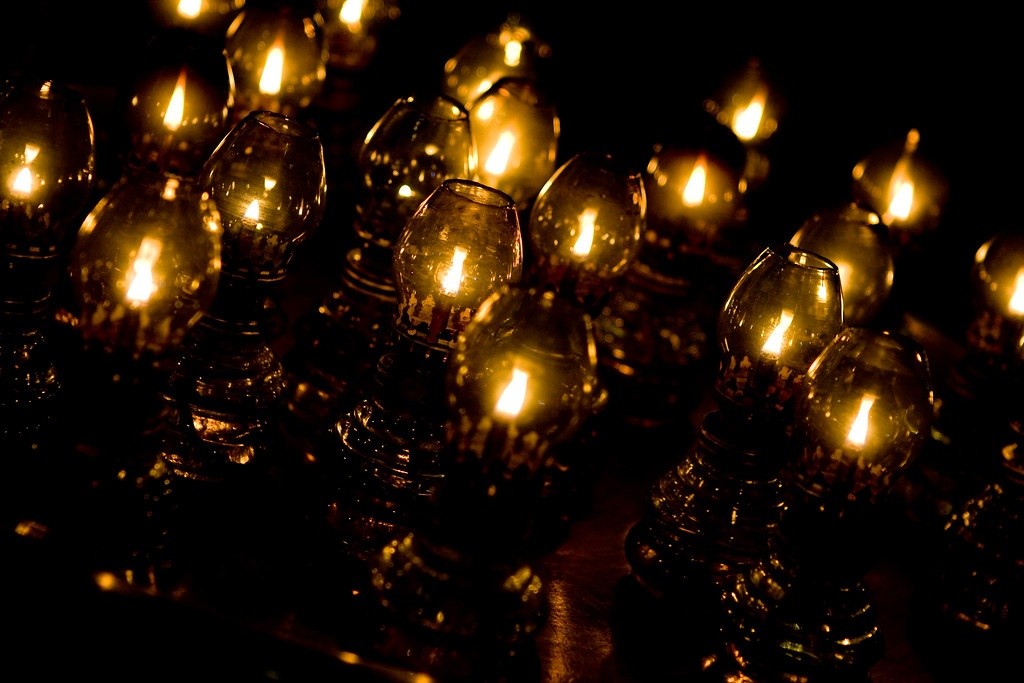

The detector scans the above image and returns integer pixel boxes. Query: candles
[479,369,531,474]
[746,310,797,397]
[562,209,599,295]
[831,392,880,501]
[234,176,276,265]
[117,232,168,347]
[430,242,470,339]
